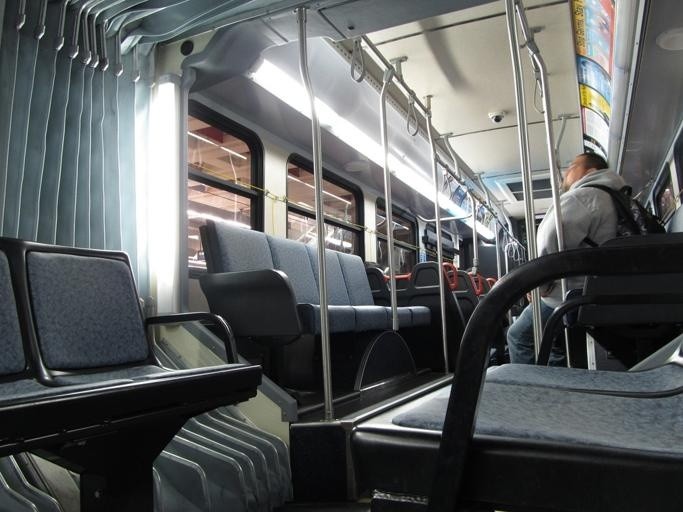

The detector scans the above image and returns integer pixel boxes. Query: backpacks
[579,184,666,248]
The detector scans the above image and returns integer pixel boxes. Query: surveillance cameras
[488,111,507,123]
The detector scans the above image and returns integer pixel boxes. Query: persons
[504,152,632,365]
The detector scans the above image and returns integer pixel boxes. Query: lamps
[149,74,183,330]
[247,55,495,240]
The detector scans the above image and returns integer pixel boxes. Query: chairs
[1,231,267,484]
[338,204,682,512]
[196,216,432,400]
